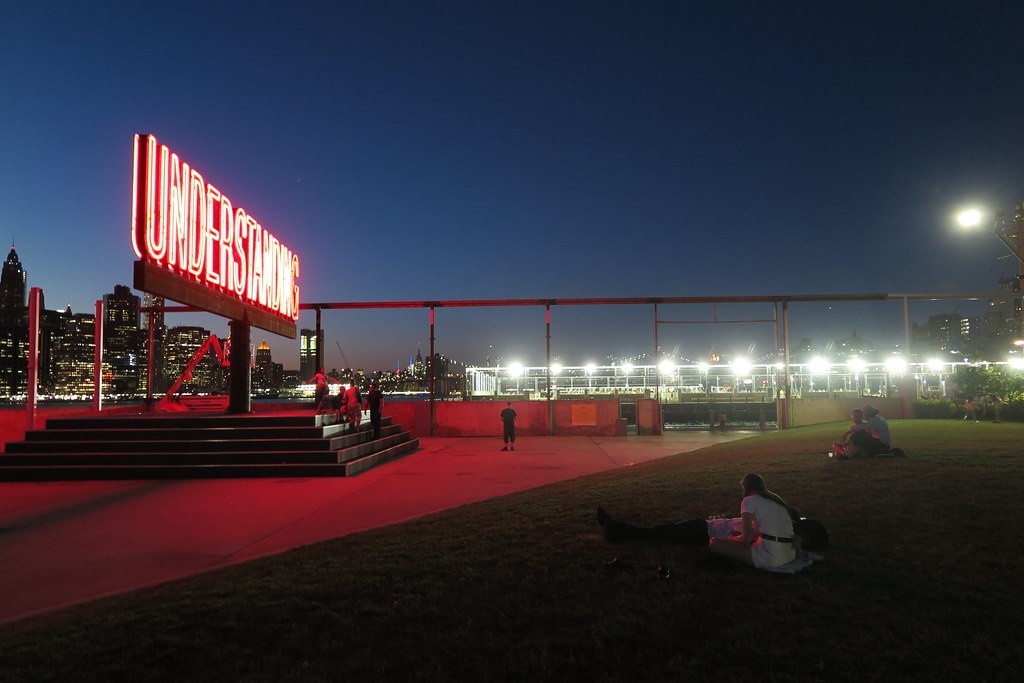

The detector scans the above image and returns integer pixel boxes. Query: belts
[762,533,794,542]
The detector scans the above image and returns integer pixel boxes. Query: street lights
[957,206,1024,265]
[512,355,1024,391]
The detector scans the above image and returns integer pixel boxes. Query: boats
[292,383,359,398]
[251,386,280,399]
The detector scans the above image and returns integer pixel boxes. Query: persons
[991,397,1001,423]
[338,379,363,433]
[838,403,891,460]
[500,401,518,451]
[961,396,981,423]
[306,367,337,409]
[364,383,384,440]
[596,472,828,570]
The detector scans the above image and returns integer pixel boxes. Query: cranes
[335,341,349,369]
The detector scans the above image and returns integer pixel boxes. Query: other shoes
[597,506,624,545]
[836,454,853,461]
[501,447,508,451]
[511,447,515,451]
[356,428,359,433]
